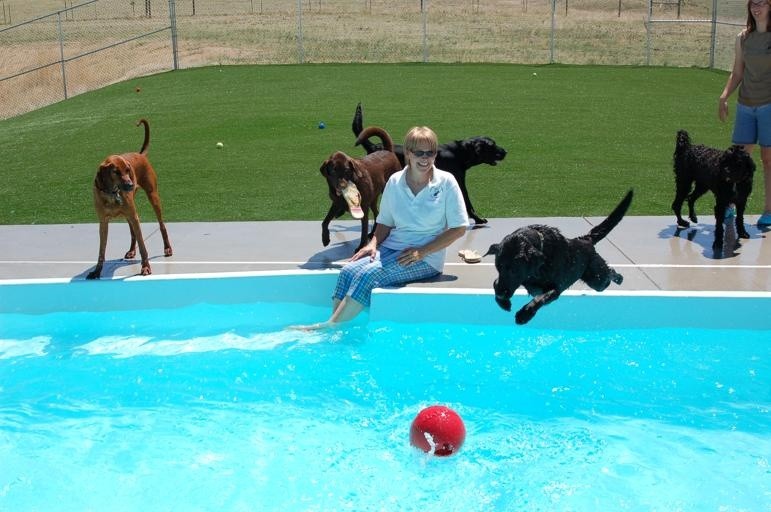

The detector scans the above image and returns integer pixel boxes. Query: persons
[719,0,771,227]
[295,124,469,330]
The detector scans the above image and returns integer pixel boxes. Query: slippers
[341,180,364,219]
[458,249,481,262]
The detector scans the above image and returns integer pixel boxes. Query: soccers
[411,405,465,455]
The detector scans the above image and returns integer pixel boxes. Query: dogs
[320,126,403,254]
[483,189,635,325]
[86,118,173,280]
[672,130,758,259]
[352,102,509,224]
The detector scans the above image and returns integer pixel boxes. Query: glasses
[749,1,769,7]
[409,149,436,157]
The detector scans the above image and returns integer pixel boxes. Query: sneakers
[724,207,734,218]
[758,210,771,225]
